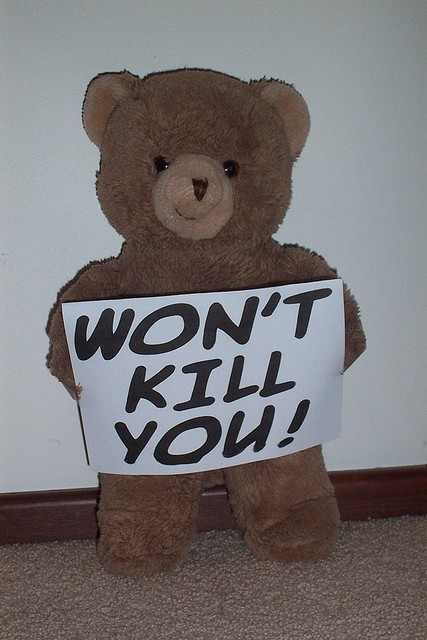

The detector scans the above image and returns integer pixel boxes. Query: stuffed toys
[42,66,368,577]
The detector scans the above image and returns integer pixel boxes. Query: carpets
[0,514,426,639]
